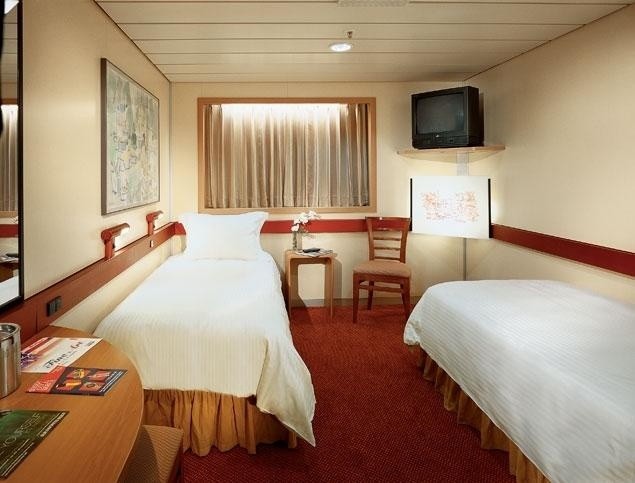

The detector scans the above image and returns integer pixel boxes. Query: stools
[121,425,184,483]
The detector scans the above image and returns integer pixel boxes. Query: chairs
[353,216,411,322]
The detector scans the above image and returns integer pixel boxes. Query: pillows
[179,211,268,259]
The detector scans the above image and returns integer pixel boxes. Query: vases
[292,230,302,252]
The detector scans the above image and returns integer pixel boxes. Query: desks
[0,326,144,483]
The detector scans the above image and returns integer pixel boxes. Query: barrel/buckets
[0,322,21,401]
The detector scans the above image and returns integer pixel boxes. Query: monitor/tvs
[411,85,480,149]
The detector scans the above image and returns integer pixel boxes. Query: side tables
[284,249,334,320]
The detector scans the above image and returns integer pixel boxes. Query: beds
[90,254,317,458]
[403,280,635,483]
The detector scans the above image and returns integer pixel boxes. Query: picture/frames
[100,57,159,216]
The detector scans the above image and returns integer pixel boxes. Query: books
[25,365,127,396]
[296,246,334,258]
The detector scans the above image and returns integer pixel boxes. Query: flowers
[290,210,321,232]
